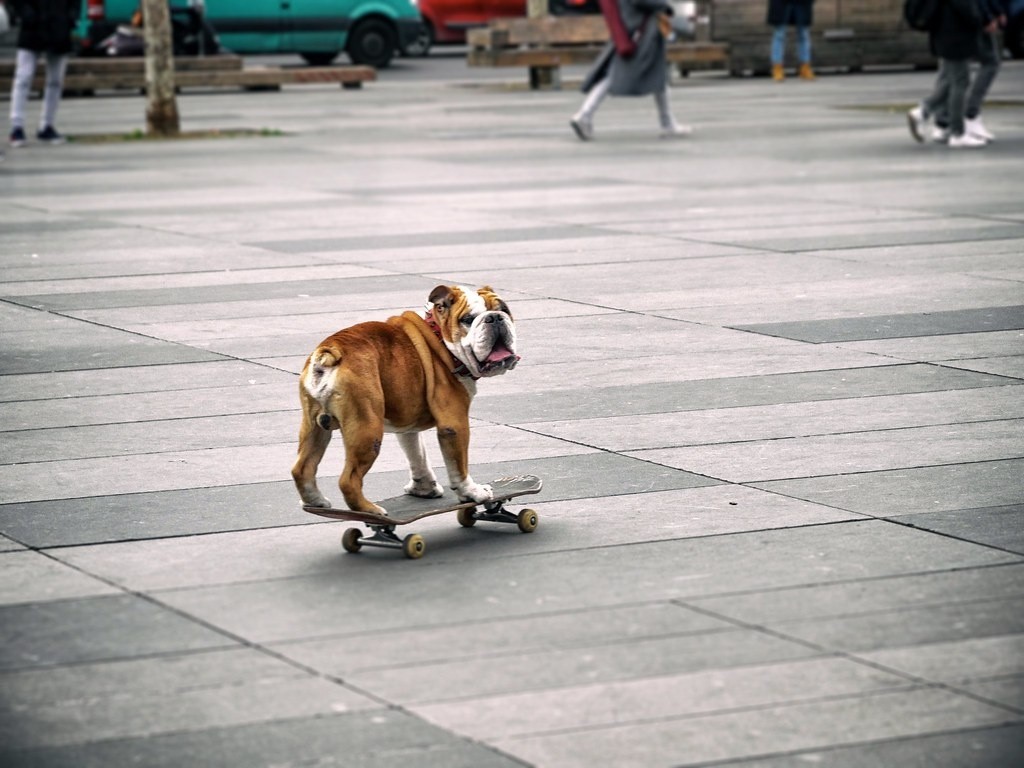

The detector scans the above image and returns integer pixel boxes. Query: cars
[409,0,612,53]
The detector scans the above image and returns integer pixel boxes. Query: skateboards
[302,473,543,558]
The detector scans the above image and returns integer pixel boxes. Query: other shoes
[569,115,595,140]
[906,104,994,149]
[660,123,692,139]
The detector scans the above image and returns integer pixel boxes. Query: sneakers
[36,125,69,145]
[8,128,31,149]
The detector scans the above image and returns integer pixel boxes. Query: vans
[53,0,425,69]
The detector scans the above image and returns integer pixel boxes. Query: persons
[905,0,992,148]
[933,0,1009,143]
[765,0,818,85]
[568,0,695,145]
[1,1,83,151]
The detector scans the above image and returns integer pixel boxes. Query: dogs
[291,281,518,519]
